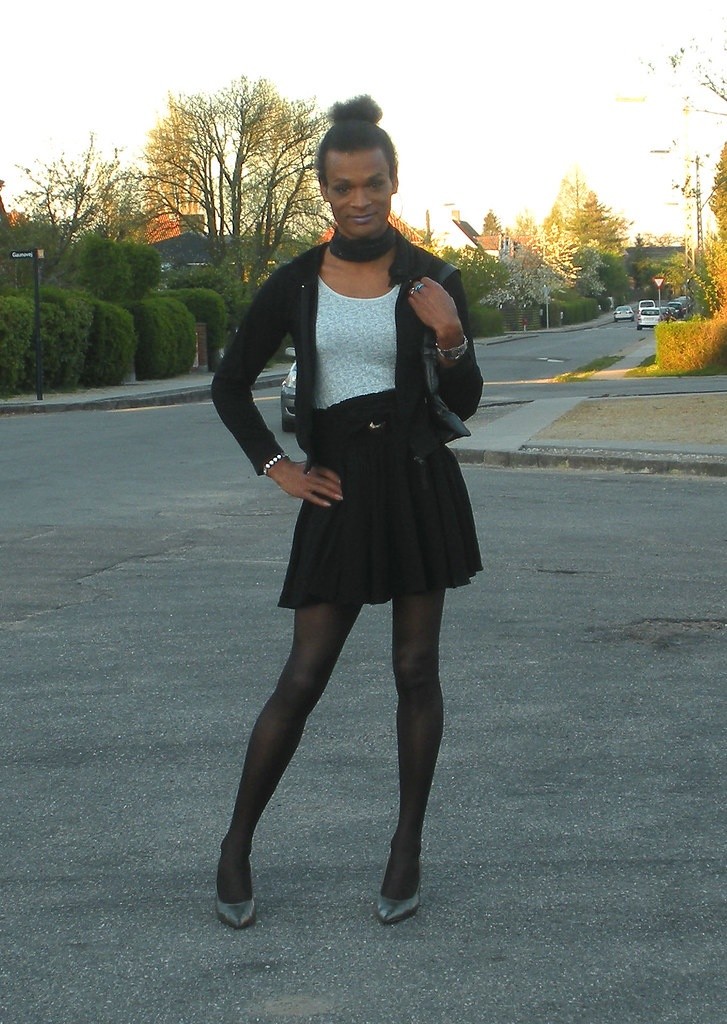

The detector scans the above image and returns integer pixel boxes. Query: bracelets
[262,453,290,477]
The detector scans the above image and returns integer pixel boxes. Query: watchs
[434,335,469,362]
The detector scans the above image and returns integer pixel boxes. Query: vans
[638,299,655,316]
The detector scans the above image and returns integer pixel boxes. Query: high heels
[214,861,256,928]
[374,867,422,924]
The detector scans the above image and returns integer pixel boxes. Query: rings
[410,289,416,295]
[416,284,425,290]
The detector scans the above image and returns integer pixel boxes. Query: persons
[212,93,485,929]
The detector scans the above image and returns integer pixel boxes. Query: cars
[637,307,660,331]
[280,346,297,433]
[613,305,634,322]
[659,296,690,322]
